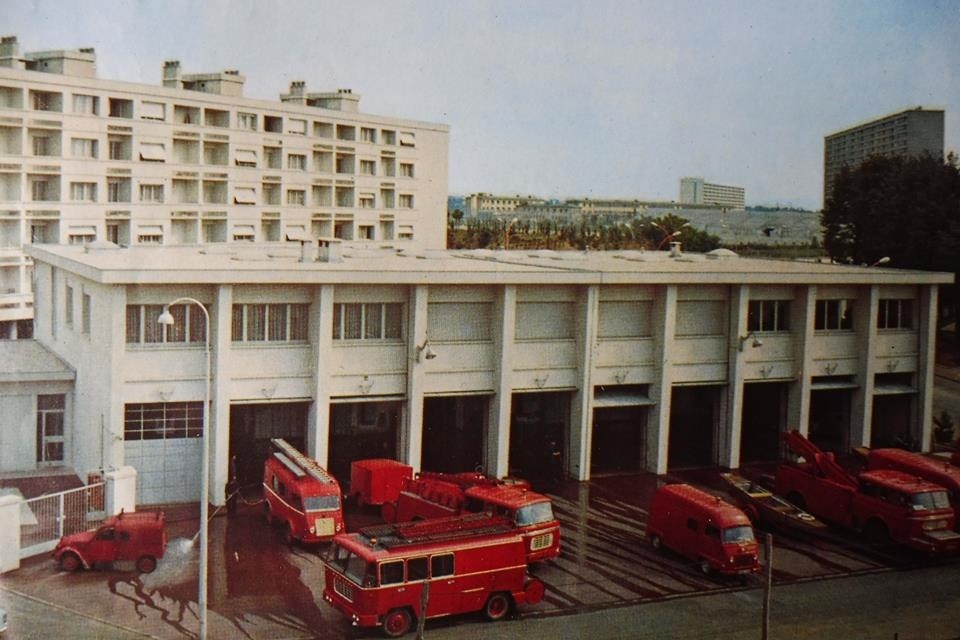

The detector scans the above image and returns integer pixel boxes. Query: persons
[224,476,239,518]
[342,490,364,532]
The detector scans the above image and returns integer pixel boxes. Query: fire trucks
[646,484,762,578]
[264,437,562,636]
[773,427,960,564]
[52,509,169,575]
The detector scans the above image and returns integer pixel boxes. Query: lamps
[738,332,764,352]
[416,335,438,364]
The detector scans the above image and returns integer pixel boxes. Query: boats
[719,472,828,534]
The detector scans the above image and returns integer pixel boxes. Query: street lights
[871,256,891,267]
[651,221,691,252]
[158,297,213,640]
[496,217,518,251]
